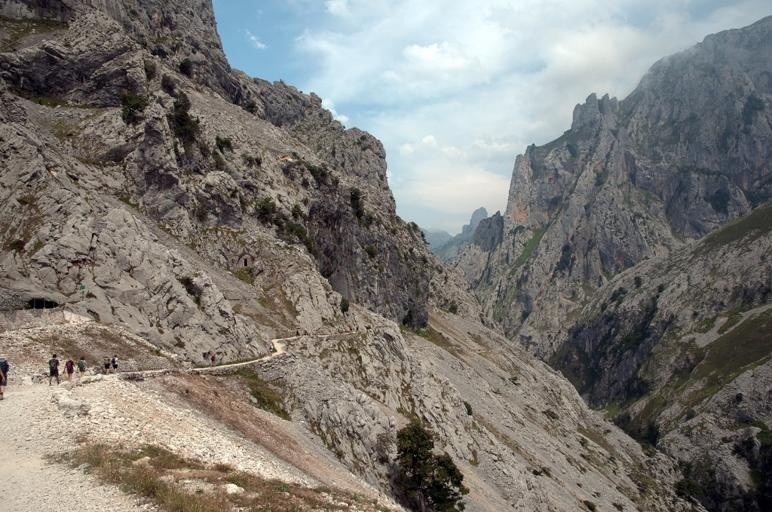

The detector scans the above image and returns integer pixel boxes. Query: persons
[63,356,76,381]
[111,354,118,373]
[104,359,111,375]
[0,357,9,400]
[48,354,59,386]
[78,357,87,379]
